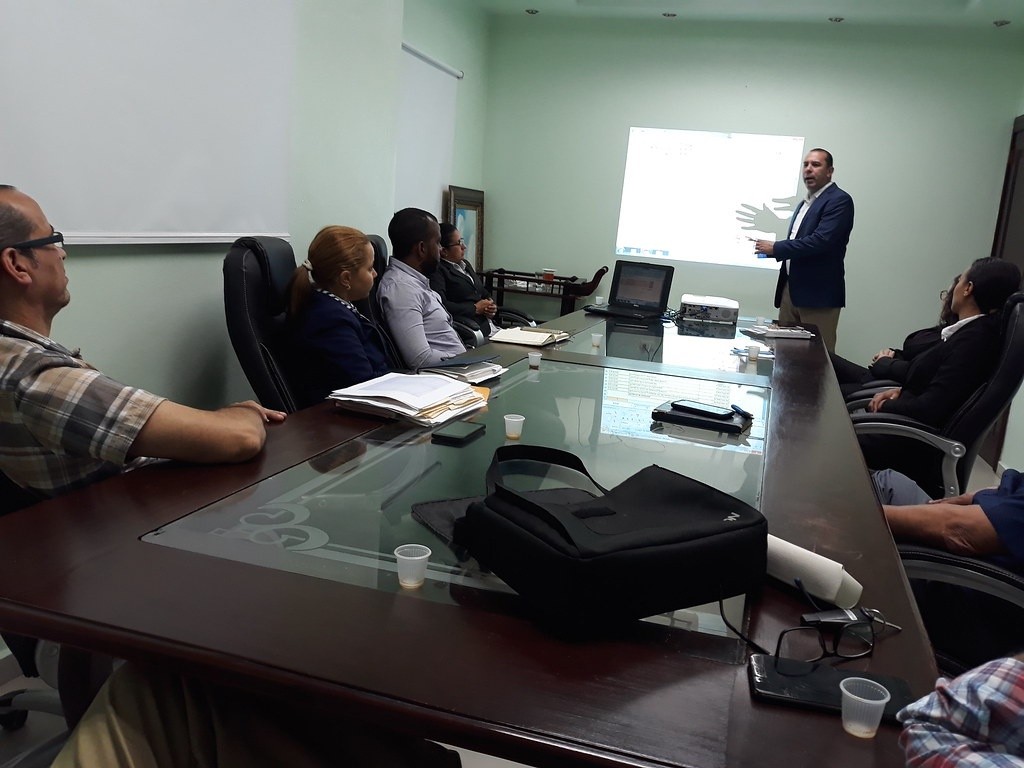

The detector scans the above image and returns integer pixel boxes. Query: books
[754,326,811,338]
[417,354,502,384]
[489,326,570,346]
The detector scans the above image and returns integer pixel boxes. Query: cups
[591,333,603,348]
[542,268,557,282]
[394,543,432,589]
[840,676,891,739]
[528,352,542,370]
[748,346,760,361]
[596,296,603,304]
[504,413,525,440]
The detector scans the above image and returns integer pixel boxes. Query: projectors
[679,293,739,324]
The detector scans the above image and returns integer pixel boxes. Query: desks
[0,308,955,768]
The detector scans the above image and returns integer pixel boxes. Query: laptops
[585,260,675,320]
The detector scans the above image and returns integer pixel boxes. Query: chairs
[359,231,396,365]
[849,293,1024,507]
[900,545,1024,676]
[223,233,305,415]
[0,469,104,732]
[451,287,540,347]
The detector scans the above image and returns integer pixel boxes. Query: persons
[376,207,466,367]
[874,469,1024,569]
[755,148,855,352]
[431,222,503,341]
[897,653,1024,768]
[0,185,287,676]
[50,658,461,768]
[829,257,1022,456]
[286,225,396,393]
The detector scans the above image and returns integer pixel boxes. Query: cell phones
[432,419,486,443]
[749,653,920,724]
[671,399,735,420]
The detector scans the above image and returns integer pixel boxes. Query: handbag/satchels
[411,444,769,634]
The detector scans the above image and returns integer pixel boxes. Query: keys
[861,606,902,630]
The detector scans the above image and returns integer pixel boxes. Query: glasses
[1,232,65,253]
[720,578,875,662]
[940,290,949,300]
[445,236,465,250]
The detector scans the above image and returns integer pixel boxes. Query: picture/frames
[448,182,486,272]
